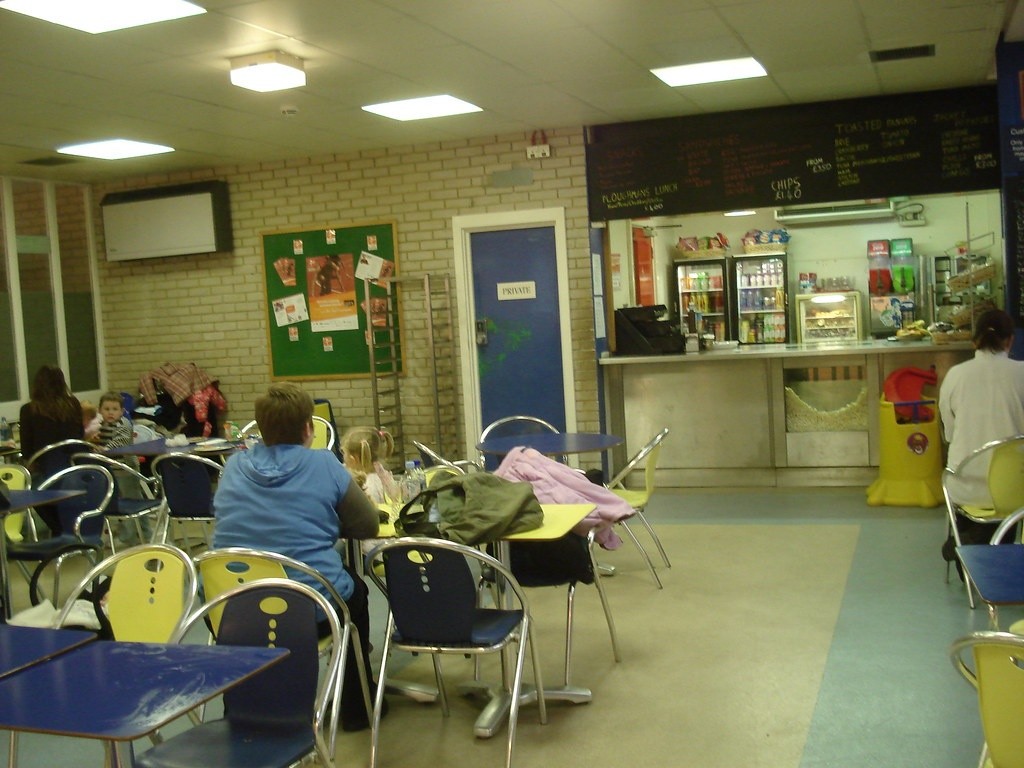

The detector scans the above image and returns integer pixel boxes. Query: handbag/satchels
[394,472,544,547]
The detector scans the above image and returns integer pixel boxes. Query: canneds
[741,260,784,287]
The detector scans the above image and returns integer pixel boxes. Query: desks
[391,503,599,740]
[0,489,87,622]
[0,640,292,768]
[344,503,439,707]
[103,437,213,550]
[0,626,98,680]
[189,438,265,456]
[475,433,626,577]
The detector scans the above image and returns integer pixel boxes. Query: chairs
[947,631,1024,768]
[941,434,1024,609]
[0,415,672,768]
[954,507,1024,632]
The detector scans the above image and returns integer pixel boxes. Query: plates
[187,437,205,443]
[194,447,231,451]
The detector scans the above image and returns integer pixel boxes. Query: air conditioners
[774,202,898,226]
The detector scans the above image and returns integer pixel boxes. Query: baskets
[930,263,997,343]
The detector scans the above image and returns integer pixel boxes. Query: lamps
[229,49,307,93]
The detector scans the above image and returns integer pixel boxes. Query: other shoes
[342,695,387,731]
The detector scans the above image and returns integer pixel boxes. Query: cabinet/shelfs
[943,231,997,333]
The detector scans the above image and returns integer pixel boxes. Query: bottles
[741,313,785,343]
[244,435,259,450]
[401,461,421,515]
[223,421,240,444]
[12,423,21,449]
[414,461,427,504]
[683,292,724,314]
[2,417,11,442]
[742,259,784,286]
[740,289,785,310]
[686,271,723,290]
[820,272,850,293]
[695,314,725,342]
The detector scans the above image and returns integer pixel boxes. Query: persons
[196,382,387,732]
[334,424,395,575]
[20,362,84,473]
[80,398,103,436]
[941,309,1024,584]
[87,390,153,545]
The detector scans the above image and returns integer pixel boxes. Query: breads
[809,308,852,318]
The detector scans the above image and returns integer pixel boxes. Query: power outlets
[899,210,925,227]
